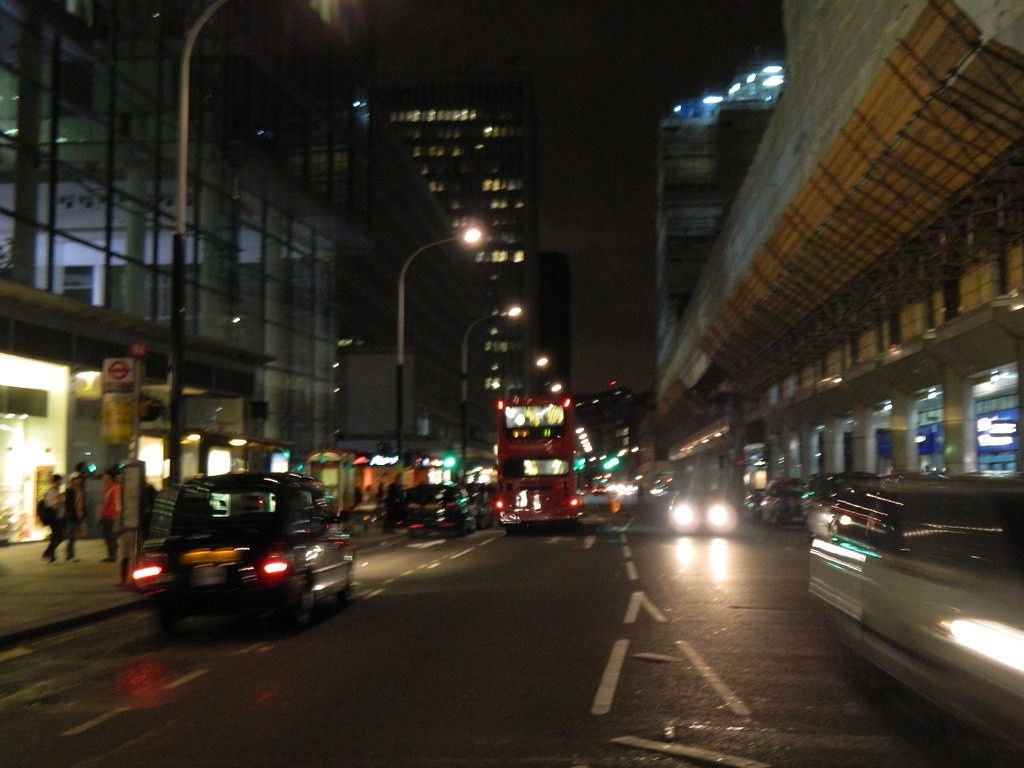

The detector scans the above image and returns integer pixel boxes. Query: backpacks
[36,500,56,526]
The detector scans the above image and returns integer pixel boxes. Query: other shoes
[103,558,115,563]
[68,558,79,563]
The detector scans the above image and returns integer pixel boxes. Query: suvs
[801,472,903,550]
[135,472,352,635]
[408,481,477,539]
[759,479,804,527]
[812,468,1024,750]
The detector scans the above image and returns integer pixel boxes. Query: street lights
[461,304,523,484]
[504,356,547,397]
[396,225,484,493]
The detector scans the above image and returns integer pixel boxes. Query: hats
[69,471,82,480]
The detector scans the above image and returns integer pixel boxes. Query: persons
[42,475,68,564]
[354,478,362,504]
[64,472,86,562]
[374,481,384,505]
[99,471,121,562]
[383,474,403,535]
[116,483,159,586]
[523,414,550,426]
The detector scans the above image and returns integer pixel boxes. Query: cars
[468,487,494,529]
[742,489,765,524]
[670,488,733,534]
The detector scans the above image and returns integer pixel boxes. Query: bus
[496,396,581,530]
